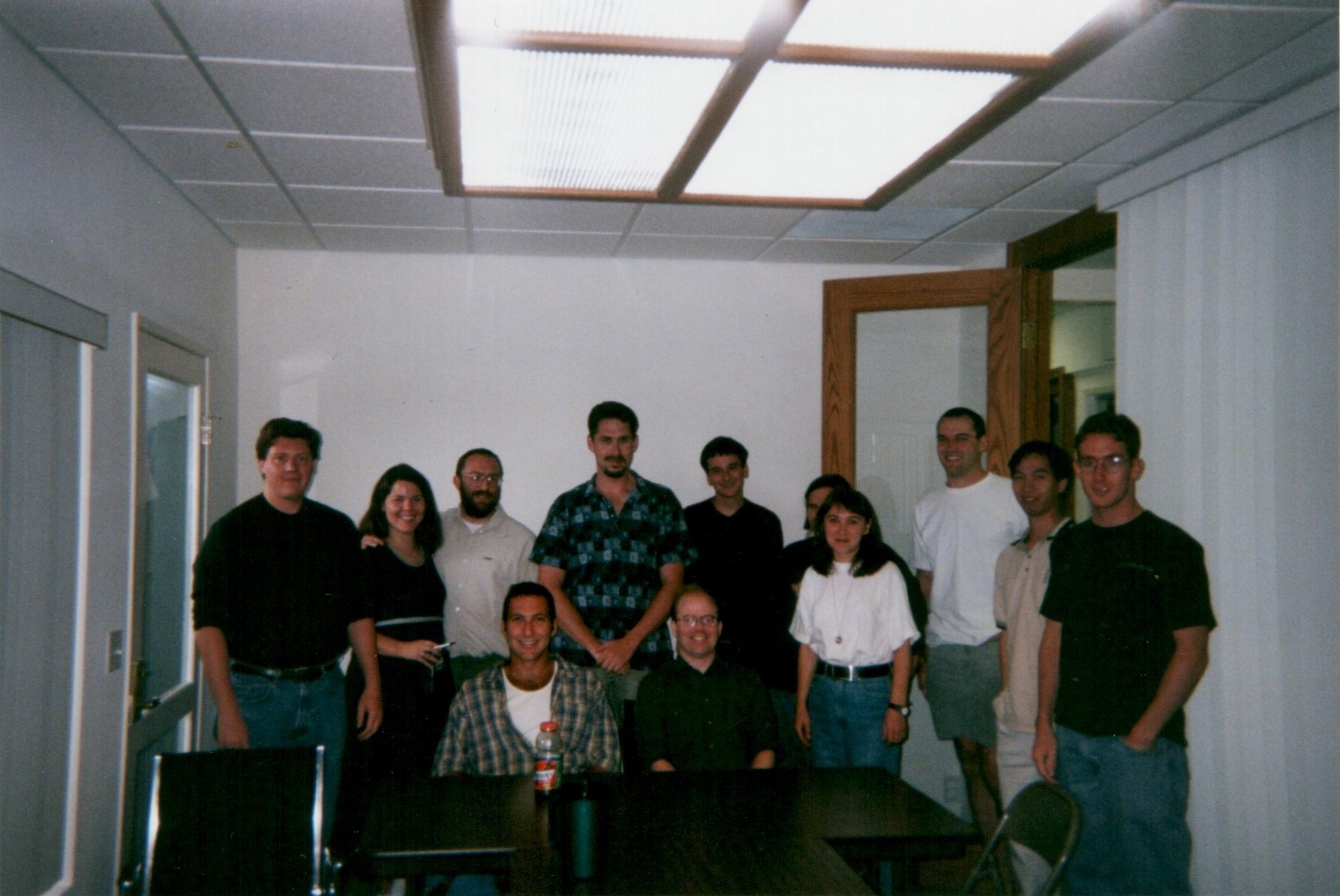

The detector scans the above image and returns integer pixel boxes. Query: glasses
[674,613,721,626]
[462,474,502,487]
[1078,455,1133,474]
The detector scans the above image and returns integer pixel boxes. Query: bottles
[534,722,563,793]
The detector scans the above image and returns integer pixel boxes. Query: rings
[422,652,426,657]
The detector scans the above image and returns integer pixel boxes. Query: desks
[375,758,981,896]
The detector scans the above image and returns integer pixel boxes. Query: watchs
[888,703,909,716]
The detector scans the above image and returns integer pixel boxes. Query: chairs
[959,781,1083,896]
[144,746,335,896]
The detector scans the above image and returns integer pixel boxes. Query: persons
[992,441,1078,896]
[191,418,381,845]
[432,582,625,896]
[788,487,918,780]
[526,401,700,699]
[911,408,1029,896]
[361,448,538,686]
[683,438,783,690]
[633,586,782,769]
[785,475,927,676]
[331,464,457,896]
[1032,410,1217,896]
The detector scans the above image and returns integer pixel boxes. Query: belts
[231,661,340,684]
[815,661,894,682]
